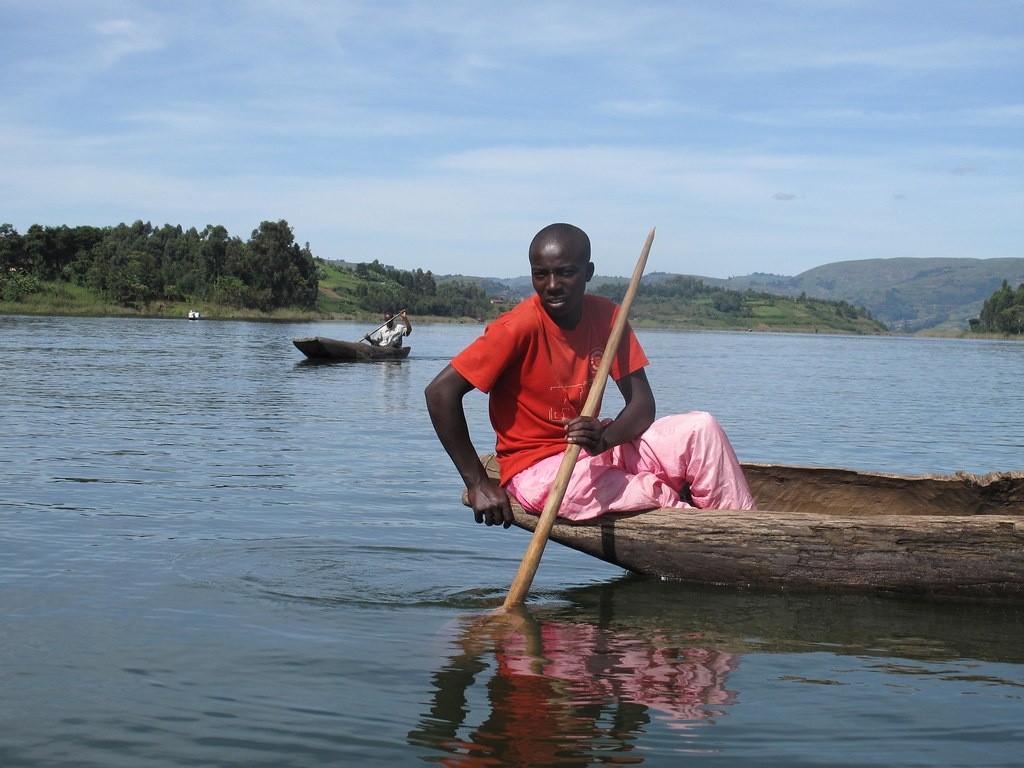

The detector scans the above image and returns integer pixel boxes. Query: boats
[463,450,1024,597]
[293,336,412,360]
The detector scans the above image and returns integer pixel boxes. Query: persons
[424,223,758,529]
[365,310,412,348]
[189,309,200,320]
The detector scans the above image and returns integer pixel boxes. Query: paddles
[357,308,406,344]
[503,226,658,608]
